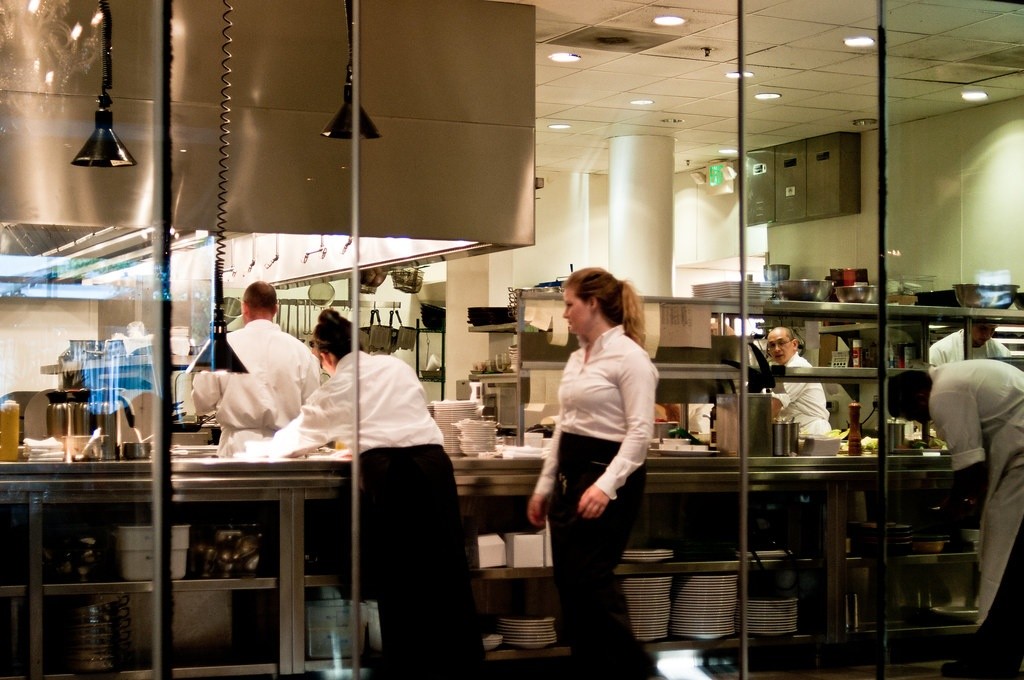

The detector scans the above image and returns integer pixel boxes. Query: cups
[772,422,801,456]
[886,423,905,454]
[473,354,507,372]
[658,444,709,450]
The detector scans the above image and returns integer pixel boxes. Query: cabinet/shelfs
[743,131,861,227]
[511,293,1024,449]
[468,322,516,383]
[0,468,984,679]
[415,319,445,403]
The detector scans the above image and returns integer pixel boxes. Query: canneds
[897,342,912,368]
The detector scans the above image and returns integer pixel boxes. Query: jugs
[44,390,136,461]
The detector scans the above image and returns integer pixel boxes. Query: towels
[21,437,65,462]
[502,445,543,459]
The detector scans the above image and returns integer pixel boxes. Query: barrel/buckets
[366,599,382,651]
[69,322,189,360]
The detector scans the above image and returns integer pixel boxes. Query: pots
[622,549,797,641]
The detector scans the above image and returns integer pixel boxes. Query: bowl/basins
[662,438,691,445]
[122,442,151,461]
[420,303,445,330]
[913,283,1024,312]
[861,525,981,555]
[70,594,131,673]
[779,280,881,304]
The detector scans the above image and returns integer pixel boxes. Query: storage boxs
[830,350,852,369]
[112,525,190,580]
[478,517,552,569]
[304,599,367,658]
[90,591,232,662]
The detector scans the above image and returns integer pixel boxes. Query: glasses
[309,339,327,348]
[766,337,797,351]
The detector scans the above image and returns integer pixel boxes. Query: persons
[928,322,1011,368]
[767,327,832,435]
[191,281,320,460]
[887,357,1024,680]
[268,308,486,680]
[528,266,664,680]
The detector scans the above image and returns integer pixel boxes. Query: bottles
[848,402,864,456]
[0,400,20,461]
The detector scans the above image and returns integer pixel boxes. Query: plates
[893,449,949,454]
[931,605,980,623]
[466,307,517,327]
[649,448,722,457]
[427,402,499,458]
[508,346,520,373]
[483,617,557,651]
[694,281,777,301]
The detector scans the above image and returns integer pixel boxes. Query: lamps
[320,0,383,139]
[691,161,737,197]
[71,0,138,168]
[186,0,250,376]
